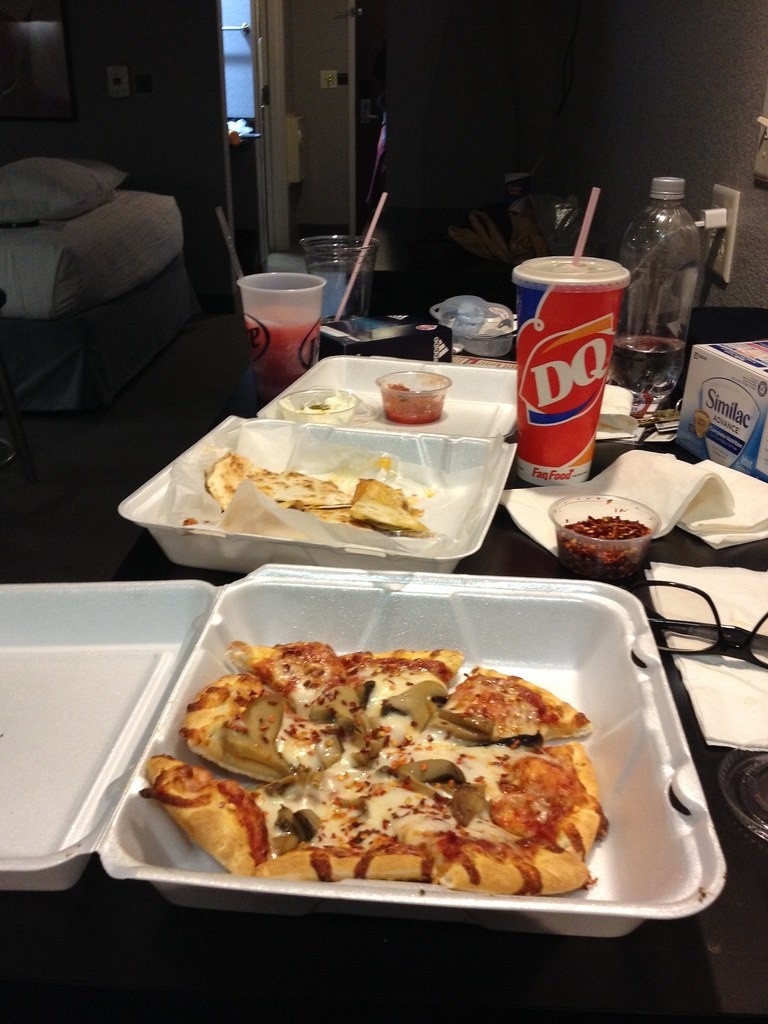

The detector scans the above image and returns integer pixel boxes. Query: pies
[204,452,429,536]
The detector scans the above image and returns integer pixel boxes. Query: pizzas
[134,641,612,891]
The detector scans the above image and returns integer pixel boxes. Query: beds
[0,189,194,415]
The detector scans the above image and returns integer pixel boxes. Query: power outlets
[320,69,340,89]
[701,184,743,284]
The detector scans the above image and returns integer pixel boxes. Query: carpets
[0,308,248,584]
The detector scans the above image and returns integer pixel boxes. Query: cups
[300,234,380,327]
[510,257,631,485]
[237,271,327,400]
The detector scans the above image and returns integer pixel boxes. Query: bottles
[615,176,704,417]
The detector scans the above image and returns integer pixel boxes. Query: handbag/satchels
[449,192,600,271]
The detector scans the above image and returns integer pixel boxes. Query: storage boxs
[676,336,768,479]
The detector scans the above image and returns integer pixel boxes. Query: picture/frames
[0,0,78,122]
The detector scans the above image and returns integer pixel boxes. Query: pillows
[0,152,130,225]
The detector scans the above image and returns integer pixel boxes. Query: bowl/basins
[379,369,452,427]
[275,387,363,431]
[548,495,659,582]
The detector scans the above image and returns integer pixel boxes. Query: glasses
[628,579,768,670]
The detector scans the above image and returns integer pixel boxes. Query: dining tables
[0,332,768,1024]
